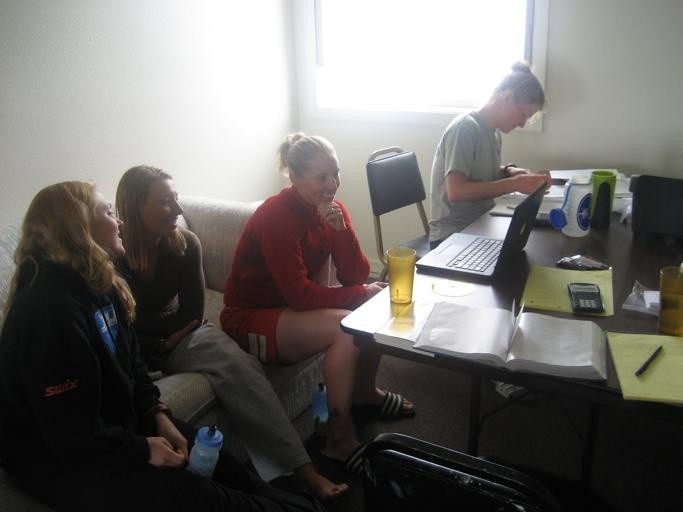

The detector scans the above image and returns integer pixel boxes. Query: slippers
[319,444,382,481]
[351,386,416,418]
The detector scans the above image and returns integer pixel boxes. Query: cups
[660,266,683,336]
[592,171,616,230]
[387,247,416,304]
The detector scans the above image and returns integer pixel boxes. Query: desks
[340,172,683,504]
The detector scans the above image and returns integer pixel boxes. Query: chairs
[366,145,433,283]
[361,432,562,512]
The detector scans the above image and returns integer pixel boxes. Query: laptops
[415,181,548,280]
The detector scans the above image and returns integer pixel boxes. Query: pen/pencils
[635,346,663,378]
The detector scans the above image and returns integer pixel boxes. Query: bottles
[188,424,224,478]
[549,175,593,236]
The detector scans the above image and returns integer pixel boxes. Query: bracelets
[501,163,518,176]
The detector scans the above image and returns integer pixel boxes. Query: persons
[427,59,554,245]
[218,133,414,476]
[113,165,349,498]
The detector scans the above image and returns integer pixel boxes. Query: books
[492,167,633,228]
[606,329,683,406]
[414,299,609,381]
